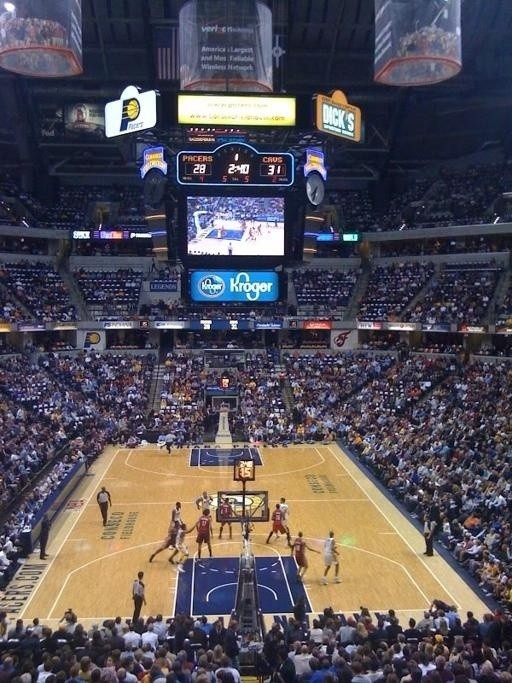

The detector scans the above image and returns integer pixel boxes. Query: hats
[435,634,443,641]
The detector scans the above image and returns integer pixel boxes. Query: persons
[1,157,512,683]
